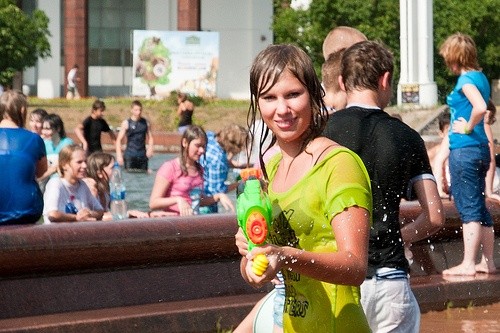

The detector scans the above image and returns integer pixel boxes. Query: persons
[233,27,445,333]
[36,113,74,194]
[29,108,48,139]
[0,90,47,225]
[66,64,81,99]
[116,100,154,173]
[177,92,194,133]
[427,99,500,202]
[189,123,255,215]
[439,31,497,276]
[81,151,178,218]
[74,99,117,157]
[148,126,235,217]
[235,43,372,333]
[42,144,105,223]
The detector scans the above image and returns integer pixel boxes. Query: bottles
[108,162,127,220]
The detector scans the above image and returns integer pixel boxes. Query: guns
[235,168,274,277]
